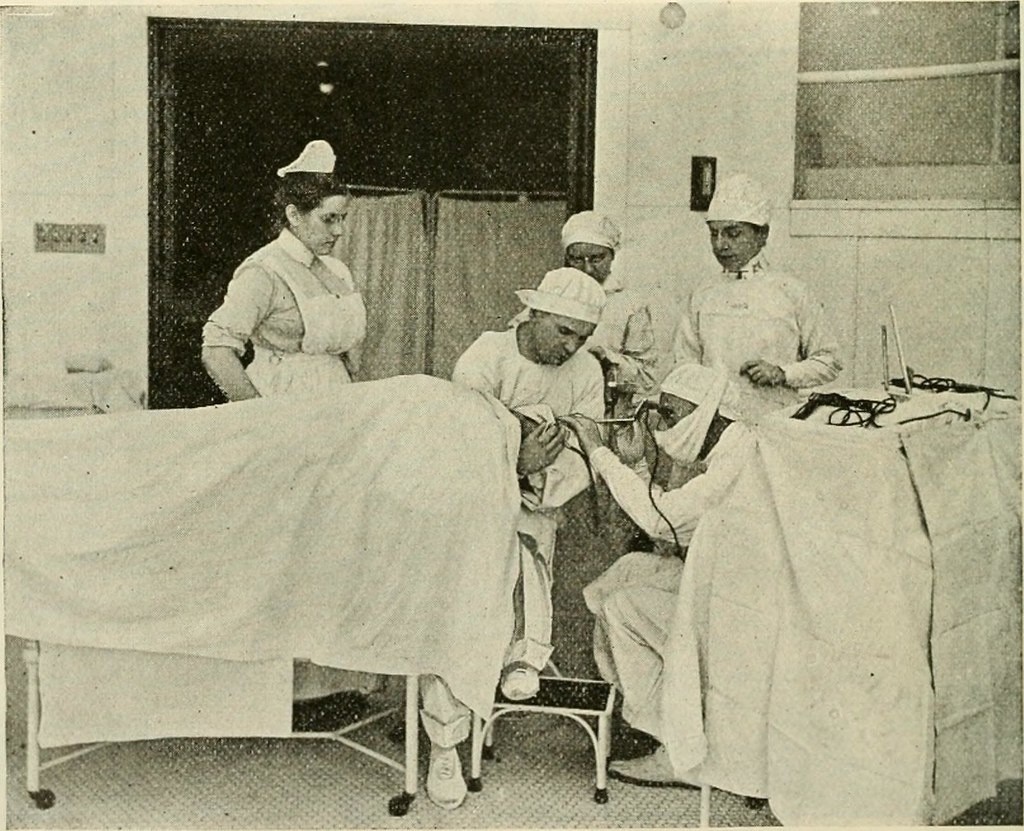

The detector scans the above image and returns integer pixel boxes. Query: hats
[514,268,606,325]
[561,211,621,251]
[277,141,336,177]
[659,363,740,421]
[704,182,771,228]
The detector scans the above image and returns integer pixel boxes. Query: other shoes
[607,743,702,789]
[500,664,539,701]
[426,742,467,810]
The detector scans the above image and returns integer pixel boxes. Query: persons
[200,139,384,734]
[558,366,738,792]
[673,172,847,412]
[507,208,661,416]
[420,267,604,810]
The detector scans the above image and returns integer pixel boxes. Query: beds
[3,374,521,831]
